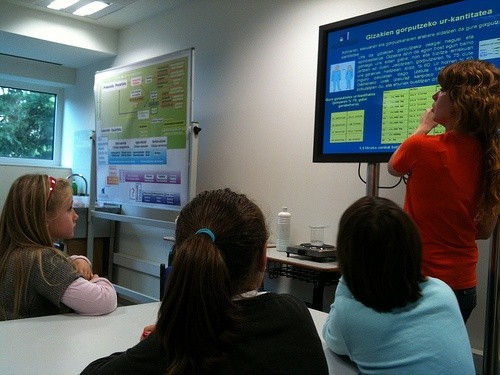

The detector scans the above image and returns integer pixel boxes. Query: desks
[159,234,277,300]
[256,247,340,314]
[0,301,362,375]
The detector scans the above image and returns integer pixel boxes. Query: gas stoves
[285,243,337,263]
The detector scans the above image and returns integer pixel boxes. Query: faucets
[66,173,88,196]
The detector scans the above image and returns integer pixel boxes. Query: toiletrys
[97,188,109,201]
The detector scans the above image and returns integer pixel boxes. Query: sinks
[61,202,122,239]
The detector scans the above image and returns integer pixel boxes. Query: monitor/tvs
[311,0,500,162]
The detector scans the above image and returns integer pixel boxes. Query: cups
[311,225,325,247]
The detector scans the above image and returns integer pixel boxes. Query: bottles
[276,207,291,252]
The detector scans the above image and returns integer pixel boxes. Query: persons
[0,172,118,317]
[324,195,477,375]
[387,57,500,324]
[80,189,329,375]
